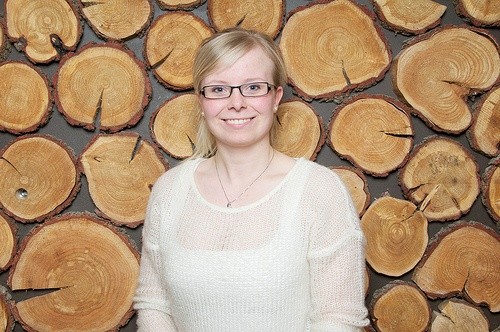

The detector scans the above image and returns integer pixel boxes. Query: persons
[131,28,370,332]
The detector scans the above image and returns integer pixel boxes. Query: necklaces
[214,148,275,208]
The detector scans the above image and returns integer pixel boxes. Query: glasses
[200,81,279,100]
